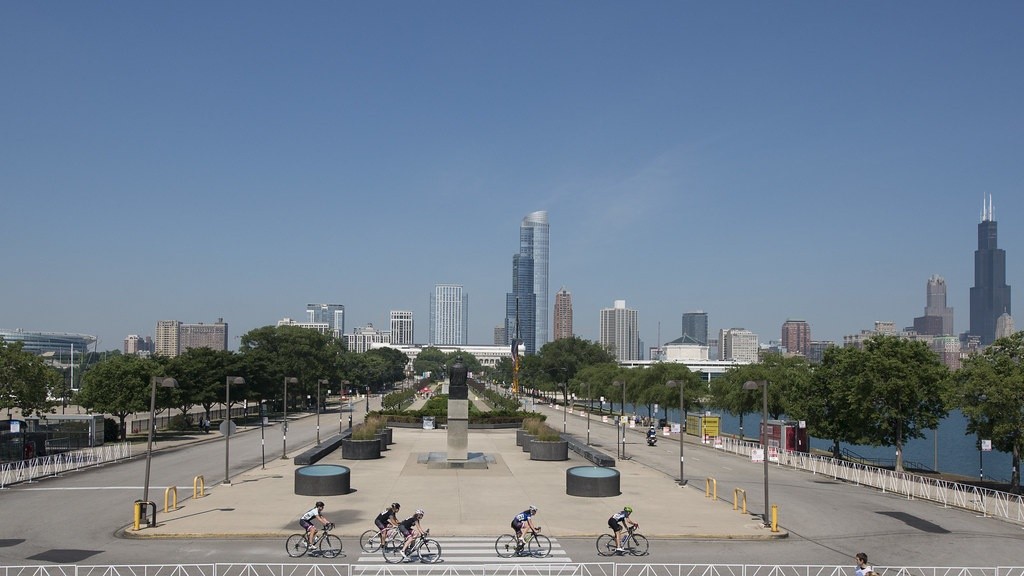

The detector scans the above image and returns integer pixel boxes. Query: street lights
[366,385,369,414]
[338,381,349,433]
[613,380,625,459]
[579,383,590,445]
[742,379,771,520]
[316,379,329,445]
[223,376,245,484]
[558,383,566,433]
[281,377,298,459]
[666,380,687,482]
[140,376,180,524]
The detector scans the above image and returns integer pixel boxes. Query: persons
[608,506,638,555]
[375,503,400,549]
[299,501,332,556]
[198,417,211,432]
[855,552,881,576]
[511,506,539,555]
[646,416,666,442]
[399,509,430,560]
[417,390,428,399]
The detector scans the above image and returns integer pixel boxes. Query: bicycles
[382,533,441,564]
[596,522,649,557]
[286,522,342,559]
[360,524,421,553]
[495,525,551,558]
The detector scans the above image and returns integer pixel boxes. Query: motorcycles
[647,436,656,446]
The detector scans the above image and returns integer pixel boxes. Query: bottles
[524,534,532,541]
[622,535,627,542]
[314,536,318,541]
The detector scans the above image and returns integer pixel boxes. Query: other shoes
[302,539,307,547]
[518,537,527,543]
[308,545,318,550]
[399,550,406,557]
[616,547,625,551]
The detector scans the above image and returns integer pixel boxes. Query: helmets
[624,507,632,512]
[529,505,537,511]
[415,509,424,514]
[316,502,324,508]
[392,503,400,508]
